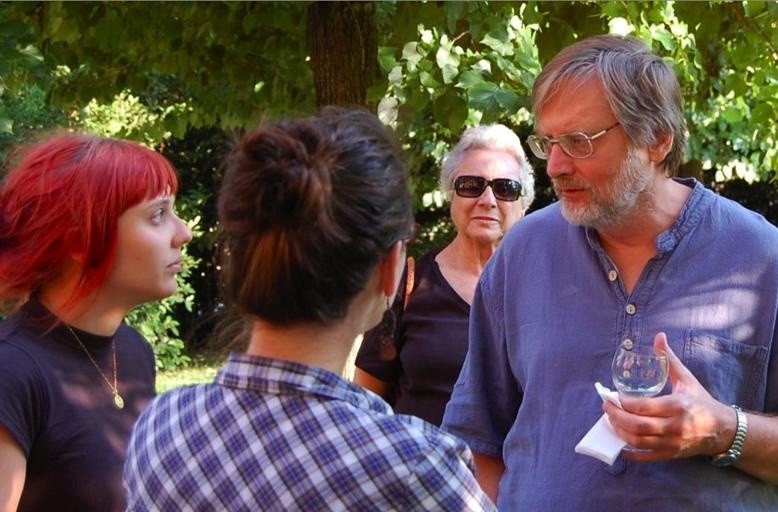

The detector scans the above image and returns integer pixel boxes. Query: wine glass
[610,344,668,454]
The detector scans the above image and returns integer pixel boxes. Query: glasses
[524,121,619,158]
[452,176,524,202]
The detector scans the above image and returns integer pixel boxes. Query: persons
[1,132,193,512]
[121,105,500,512]
[438,35,778,512]
[349,124,537,427]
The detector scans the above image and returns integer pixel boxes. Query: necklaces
[42,295,125,410]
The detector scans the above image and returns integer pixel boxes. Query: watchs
[710,405,748,468]
